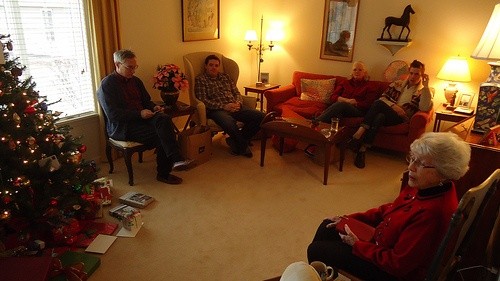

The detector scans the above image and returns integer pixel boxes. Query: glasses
[406,154,435,168]
[122,63,138,70]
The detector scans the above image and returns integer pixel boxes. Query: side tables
[244,82,279,113]
[432,107,475,138]
[154,101,195,135]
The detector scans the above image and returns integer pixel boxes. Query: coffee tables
[259,117,350,185]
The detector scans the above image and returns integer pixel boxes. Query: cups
[331,118,339,132]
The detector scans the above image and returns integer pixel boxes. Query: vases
[161,92,181,106]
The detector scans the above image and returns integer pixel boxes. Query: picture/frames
[320,0,360,63]
[261,73,269,82]
[182,0,221,42]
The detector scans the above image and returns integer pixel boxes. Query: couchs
[183,51,257,140]
[267,72,435,156]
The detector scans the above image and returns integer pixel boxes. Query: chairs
[99,101,158,187]
[324,166,500,281]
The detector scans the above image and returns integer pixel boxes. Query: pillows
[305,77,335,104]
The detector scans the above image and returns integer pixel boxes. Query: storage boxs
[176,123,215,172]
[0,177,152,281]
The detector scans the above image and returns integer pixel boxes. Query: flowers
[152,64,191,92]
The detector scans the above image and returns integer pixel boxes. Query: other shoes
[239,143,253,158]
[335,136,357,149]
[355,151,366,168]
[157,173,183,185]
[169,152,184,162]
[226,137,239,155]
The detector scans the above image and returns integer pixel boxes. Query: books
[108,204,137,221]
[119,191,153,208]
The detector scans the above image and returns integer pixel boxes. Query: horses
[379,5,416,41]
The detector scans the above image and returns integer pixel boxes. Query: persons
[196,55,264,157]
[343,61,433,168]
[95,50,183,183]
[304,63,372,156]
[307,132,472,281]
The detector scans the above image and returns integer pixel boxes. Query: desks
[453,120,500,201]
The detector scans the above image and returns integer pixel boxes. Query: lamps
[437,57,471,107]
[244,21,282,82]
[472,3,500,133]
